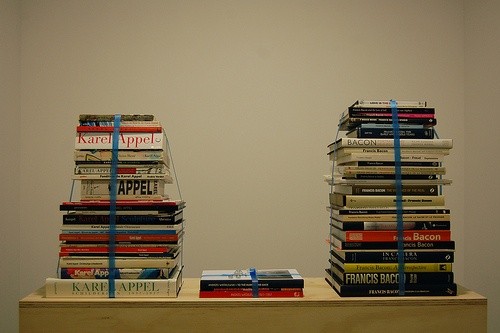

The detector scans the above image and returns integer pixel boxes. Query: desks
[17,277,488,332]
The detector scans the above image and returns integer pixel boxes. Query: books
[44,114,188,297]
[323,98,457,297]
[200,268,304,292]
[199,290,304,298]
